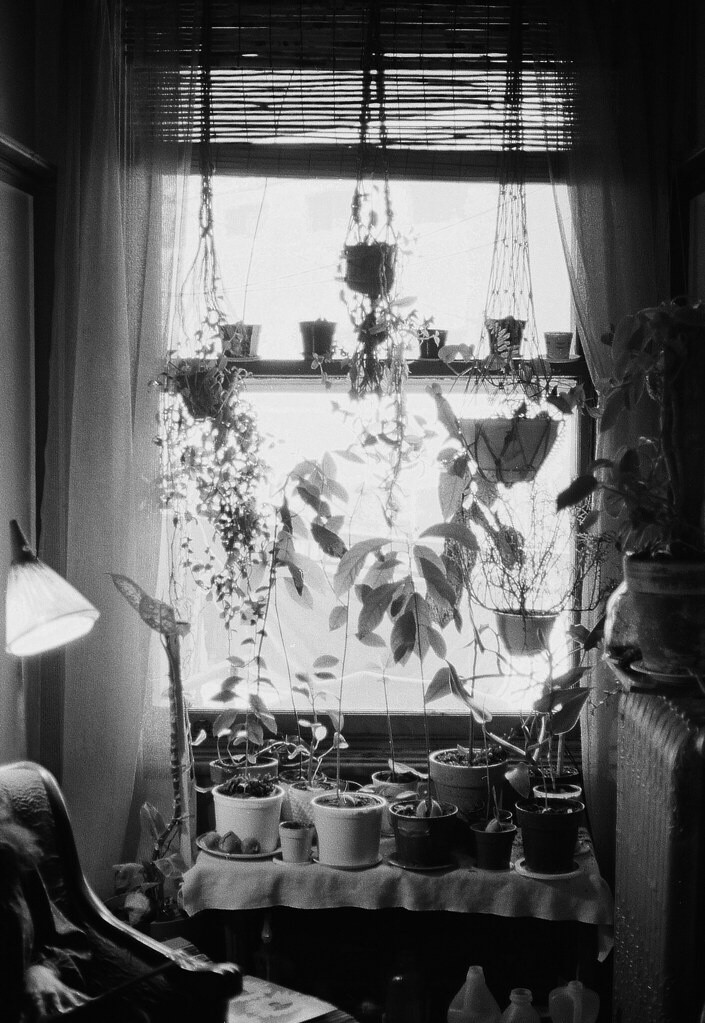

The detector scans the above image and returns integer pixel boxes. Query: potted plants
[148,240,705,873]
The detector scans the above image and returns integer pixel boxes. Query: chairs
[0,762,241,1023]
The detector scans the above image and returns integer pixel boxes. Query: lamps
[4,519,101,762]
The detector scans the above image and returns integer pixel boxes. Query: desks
[181,826,615,1023]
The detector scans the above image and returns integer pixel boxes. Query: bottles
[549,979,600,1023]
[447,965,500,1023]
[500,988,540,1023]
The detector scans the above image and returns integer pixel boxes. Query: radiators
[615,689,705,1022]
